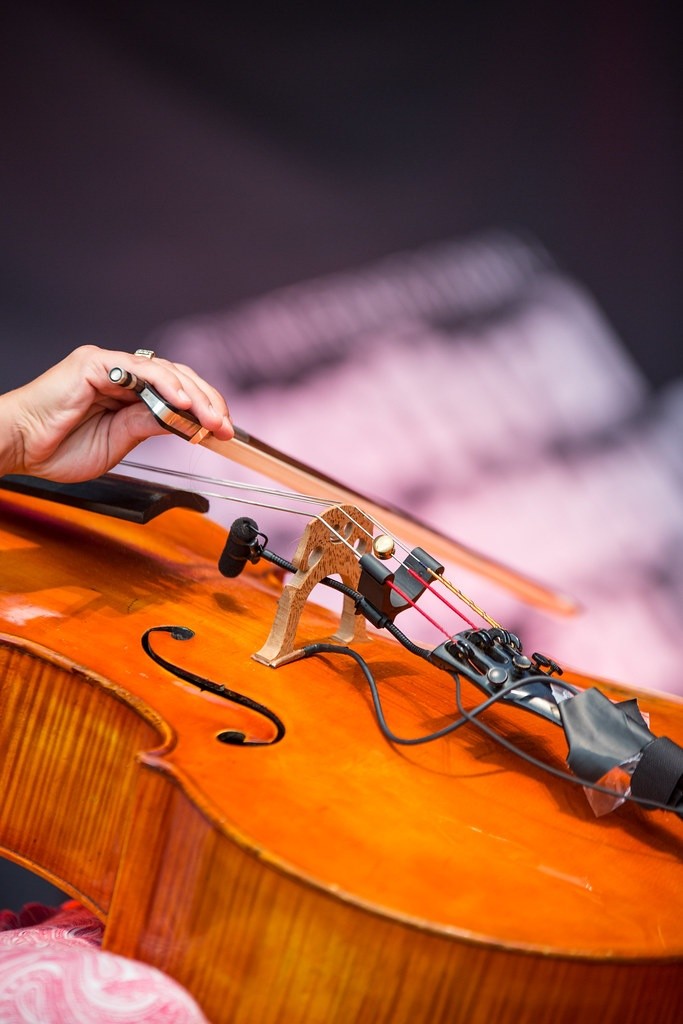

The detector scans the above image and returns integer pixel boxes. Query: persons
[1,344,236,1023]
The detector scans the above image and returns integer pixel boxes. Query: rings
[135,348,155,358]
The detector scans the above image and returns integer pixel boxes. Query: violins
[3,472,681,1023]
